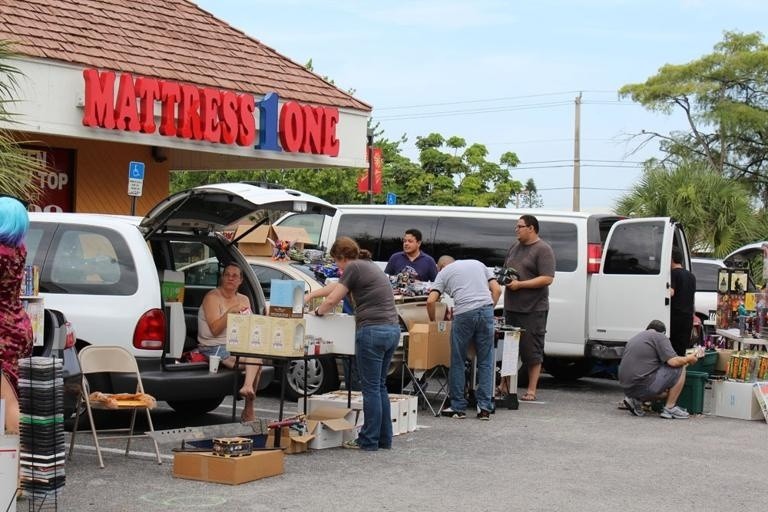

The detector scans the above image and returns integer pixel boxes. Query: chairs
[69,346,162,469]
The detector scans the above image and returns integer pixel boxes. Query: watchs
[315,307,323,316]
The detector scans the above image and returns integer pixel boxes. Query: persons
[497,215,555,400]
[0,197,34,435]
[384,230,438,281]
[197,262,262,422]
[617,319,698,419]
[304,236,400,451]
[671,247,696,355]
[427,255,502,420]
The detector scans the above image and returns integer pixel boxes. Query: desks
[717,329,768,352]
[703,320,716,336]
[403,329,525,417]
[231,350,356,421]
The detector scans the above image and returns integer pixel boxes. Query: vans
[18,177,346,435]
[266,202,698,384]
[687,239,767,326]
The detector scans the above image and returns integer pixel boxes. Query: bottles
[304,334,335,356]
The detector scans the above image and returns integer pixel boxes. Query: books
[20,265,44,347]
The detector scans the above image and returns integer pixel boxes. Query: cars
[166,251,448,404]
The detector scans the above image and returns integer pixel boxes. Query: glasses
[516,224,529,229]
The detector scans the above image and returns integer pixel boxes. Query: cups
[209,355,221,375]
[335,300,344,314]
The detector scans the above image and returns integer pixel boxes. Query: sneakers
[339,439,377,449]
[623,394,647,417]
[660,404,690,420]
[475,405,490,420]
[441,406,467,418]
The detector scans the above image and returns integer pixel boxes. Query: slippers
[519,392,538,401]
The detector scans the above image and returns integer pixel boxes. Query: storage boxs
[172,447,284,486]
[686,349,718,376]
[225,279,355,357]
[264,393,418,454]
[651,372,707,413]
[712,380,765,421]
[158,270,185,307]
[231,224,312,256]
[408,320,452,370]
